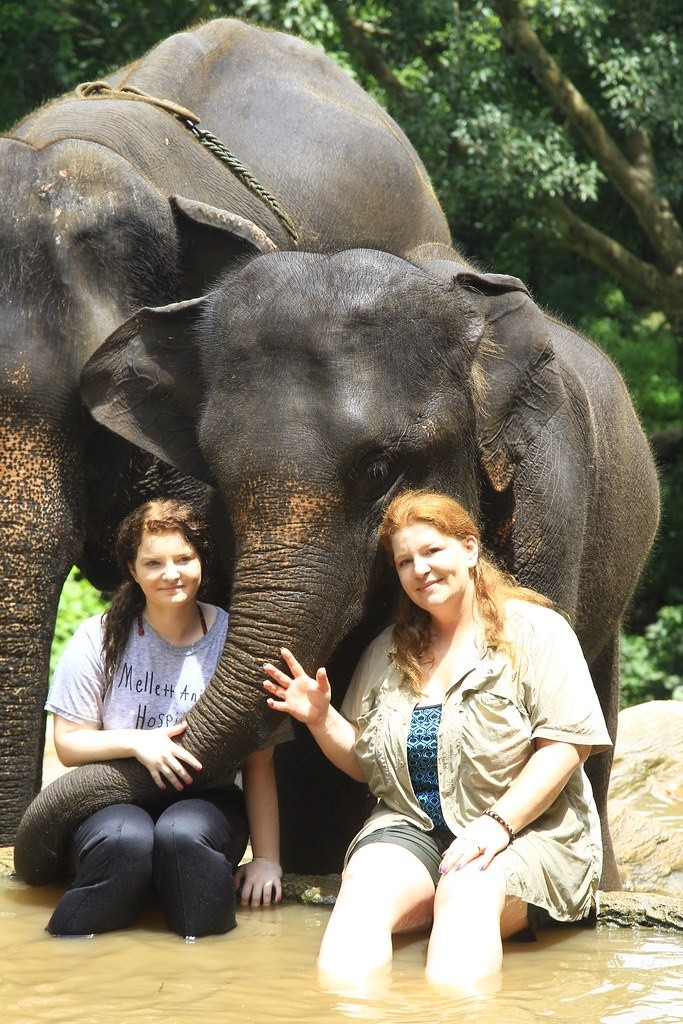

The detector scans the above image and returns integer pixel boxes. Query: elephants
[0,18,663,944]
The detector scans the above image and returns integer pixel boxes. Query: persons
[263,490,614,1001]
[45,470,296,940]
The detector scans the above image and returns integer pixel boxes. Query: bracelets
[483,810,515,847]
